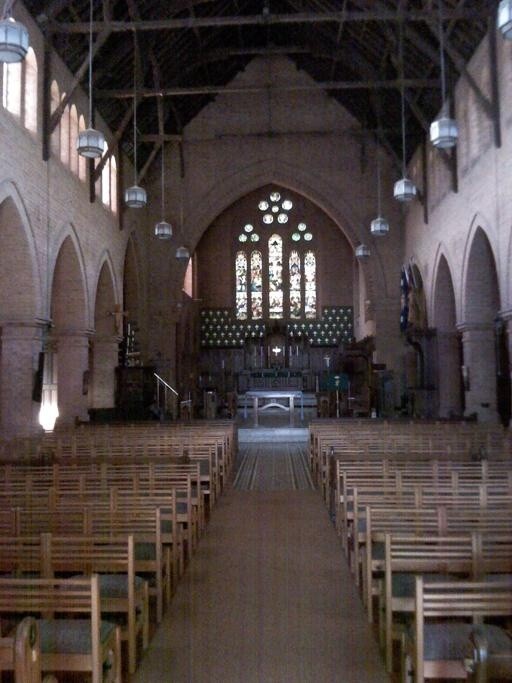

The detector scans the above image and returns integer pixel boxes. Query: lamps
[75,0,203,267]
[353,0,460,258]
[0,0,29,62]
[497,0,510,40]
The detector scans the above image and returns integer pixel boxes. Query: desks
[244,387,302,428]
